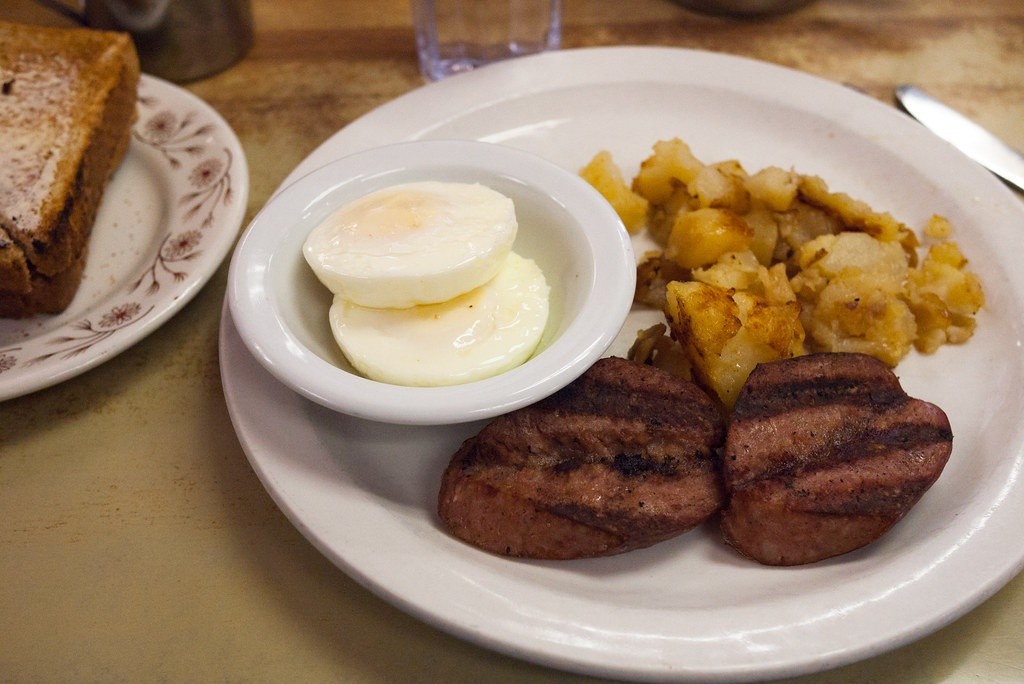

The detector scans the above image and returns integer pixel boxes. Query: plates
[220,42,1024,682]
[0,75,253,401]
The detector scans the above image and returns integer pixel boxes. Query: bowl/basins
[229,139,637,423]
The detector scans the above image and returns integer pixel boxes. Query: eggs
[303,183,550,387]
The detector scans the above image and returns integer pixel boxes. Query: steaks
[438,349,957,565]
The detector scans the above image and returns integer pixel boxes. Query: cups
[411,1,562,85]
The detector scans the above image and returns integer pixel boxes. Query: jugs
[32,0,253,85]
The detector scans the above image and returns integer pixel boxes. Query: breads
[0,22,137,320]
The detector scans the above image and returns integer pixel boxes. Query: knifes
[896,84,1024,195]
